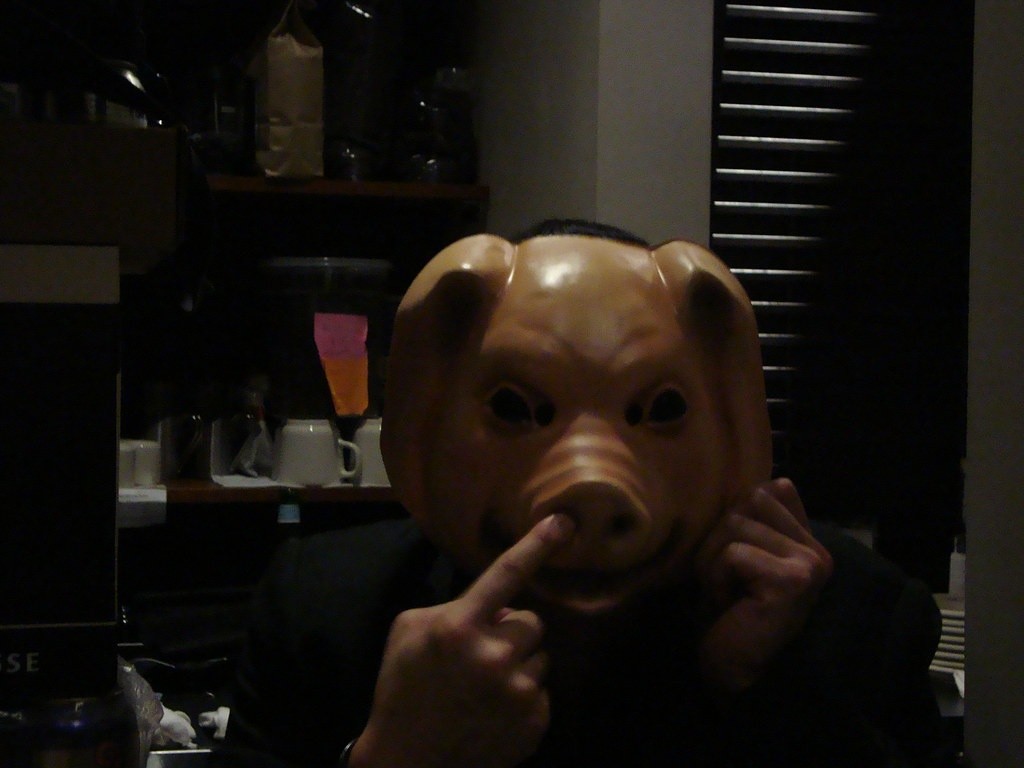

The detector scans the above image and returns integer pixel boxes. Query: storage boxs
[0,118,179,260]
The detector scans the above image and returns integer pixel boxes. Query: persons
[209,217,963,768]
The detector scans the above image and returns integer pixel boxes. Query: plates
[926,591,965,675]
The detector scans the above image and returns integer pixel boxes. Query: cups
[277,416,363,489]
[192,408,261,479]
[345,417,392,487]
[119,439,165,488]
[142,409,205,478]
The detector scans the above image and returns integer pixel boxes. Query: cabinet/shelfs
[164,175,488,503]
